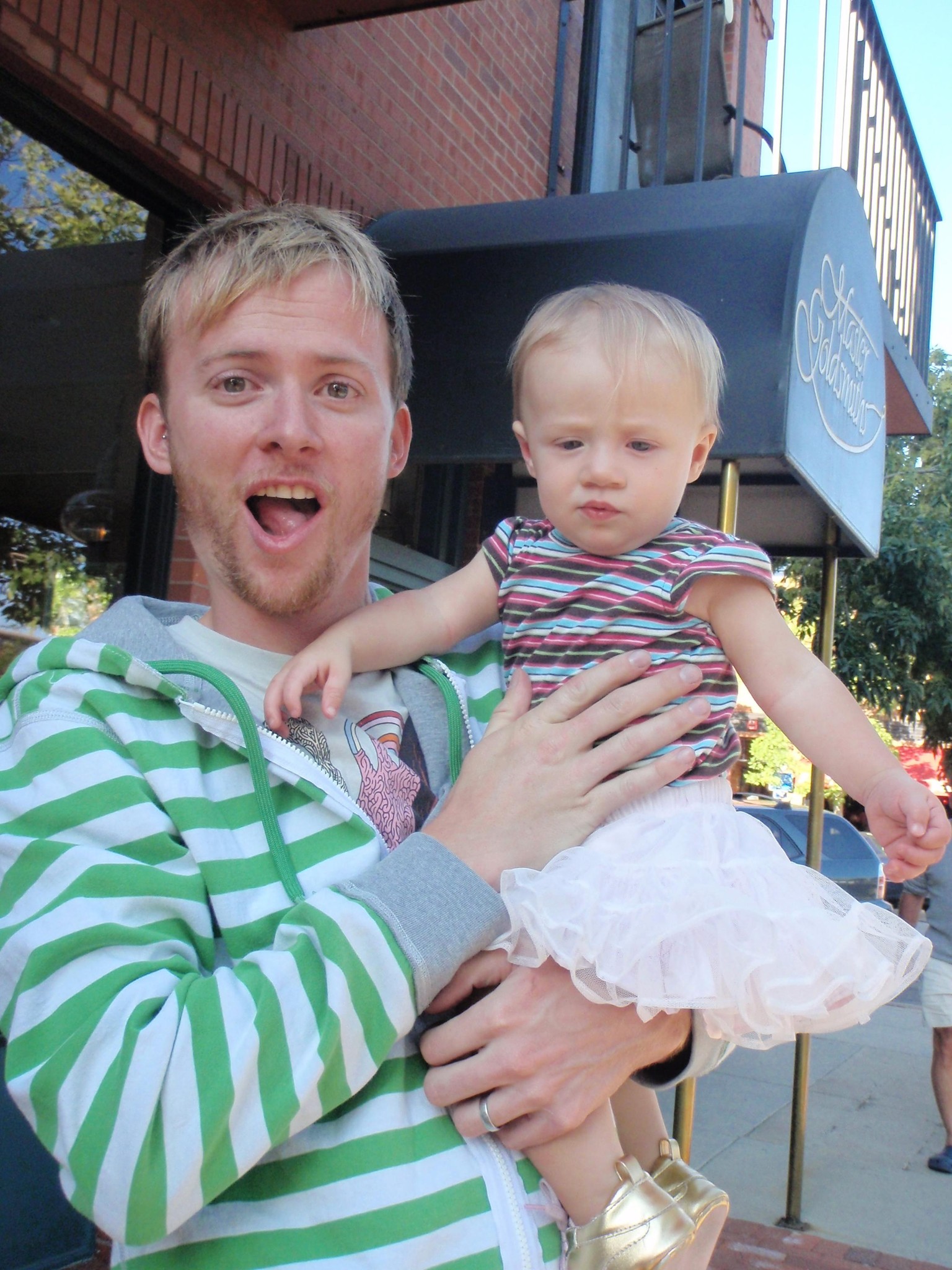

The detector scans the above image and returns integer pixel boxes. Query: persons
[898,746,952,1175]
[264,284,952,1270]
[822,793,870,832]
[1,167,714,1269]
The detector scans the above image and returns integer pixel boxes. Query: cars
[732,792,930,919]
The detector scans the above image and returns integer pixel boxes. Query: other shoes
[927,1146,952,1174]
[651,1139,731,1270]
[567,1154,696,1269]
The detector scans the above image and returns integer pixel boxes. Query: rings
[477,1096,504,1133]
